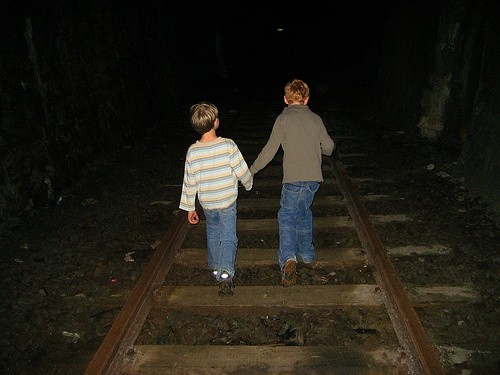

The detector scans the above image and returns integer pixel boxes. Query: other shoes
[219,271,234,295]
[281,256,297,286]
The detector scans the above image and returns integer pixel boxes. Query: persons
[248,80,336,286]
[178,104,253,299]
